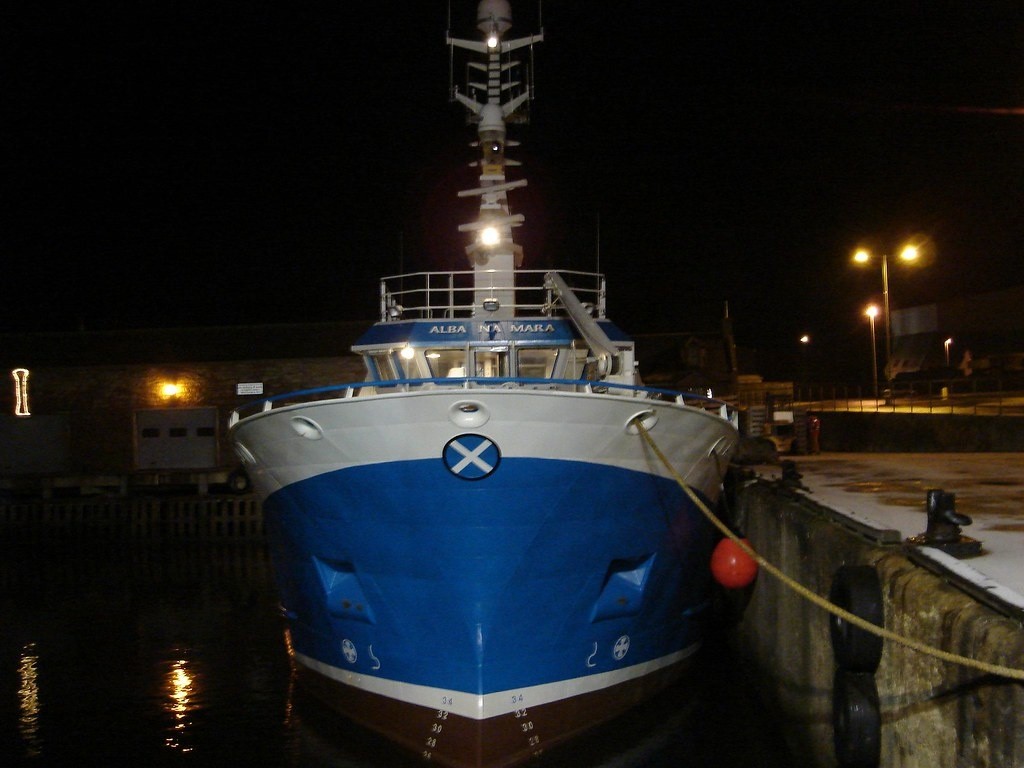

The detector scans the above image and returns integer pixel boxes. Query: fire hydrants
[807,415,820,456]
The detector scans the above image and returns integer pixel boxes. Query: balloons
[711,537,758,588]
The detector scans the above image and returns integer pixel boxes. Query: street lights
[855,245,918,404]
[864,304,882,398]
[943,338,954,372]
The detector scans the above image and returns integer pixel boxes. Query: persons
[960,350,975,377]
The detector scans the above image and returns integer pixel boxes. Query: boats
[220,0,739,719]
